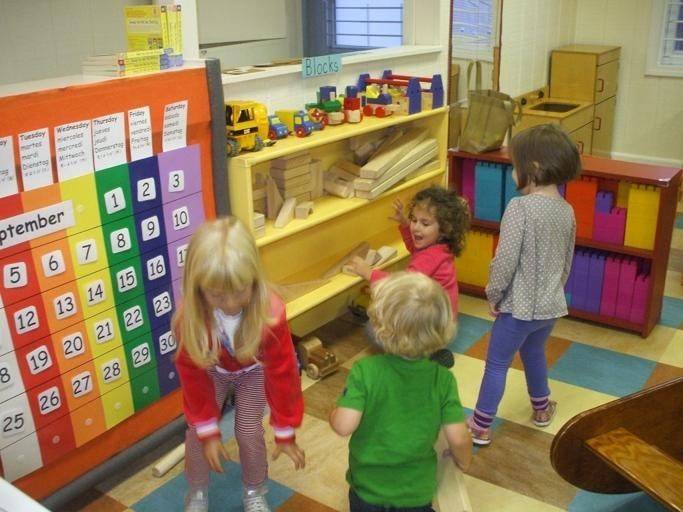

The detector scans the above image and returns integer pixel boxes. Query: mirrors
[448,2,502,149]
[193,1,411,68]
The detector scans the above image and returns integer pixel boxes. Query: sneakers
[242,483,271,512]
[183,486,210,512]
[531,400,558,427]
[463,415,493,445]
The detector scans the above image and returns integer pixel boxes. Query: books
[80,5,186,74]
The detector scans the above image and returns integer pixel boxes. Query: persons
[464,123,580,444]
[328,270,472,510]
[348,184,471,368]
[172,214,305,511]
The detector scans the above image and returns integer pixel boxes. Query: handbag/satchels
[458,59,523,155]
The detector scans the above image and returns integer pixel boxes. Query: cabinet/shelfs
[549,42,623,159]
[511,106,593,154]
[225,94,450,357]
[448,141,683,340]
